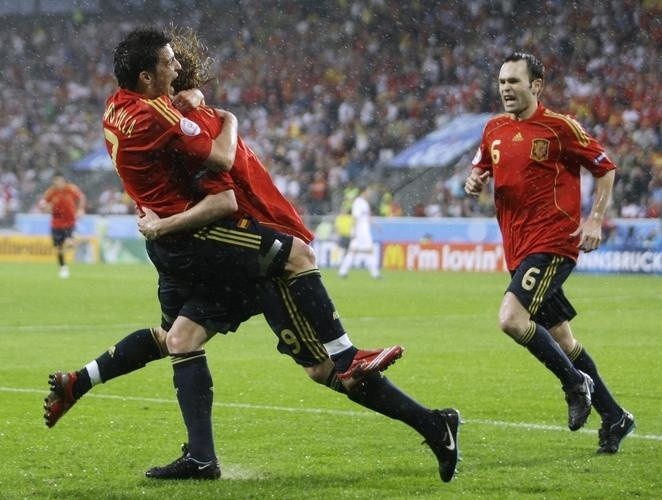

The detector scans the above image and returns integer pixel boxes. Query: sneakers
[430,407,460,483]
[561,369,595,431]
[145,442,221,480]
[335,344,405,394]
[43,369,78,428]
[595,408,637,455]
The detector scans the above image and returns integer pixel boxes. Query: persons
[338,186,380,278]
[42,25,405,429]
[1,2,662,216]
[463,51,638,456]
[131,24,466,484]
[43,174,85,279]
